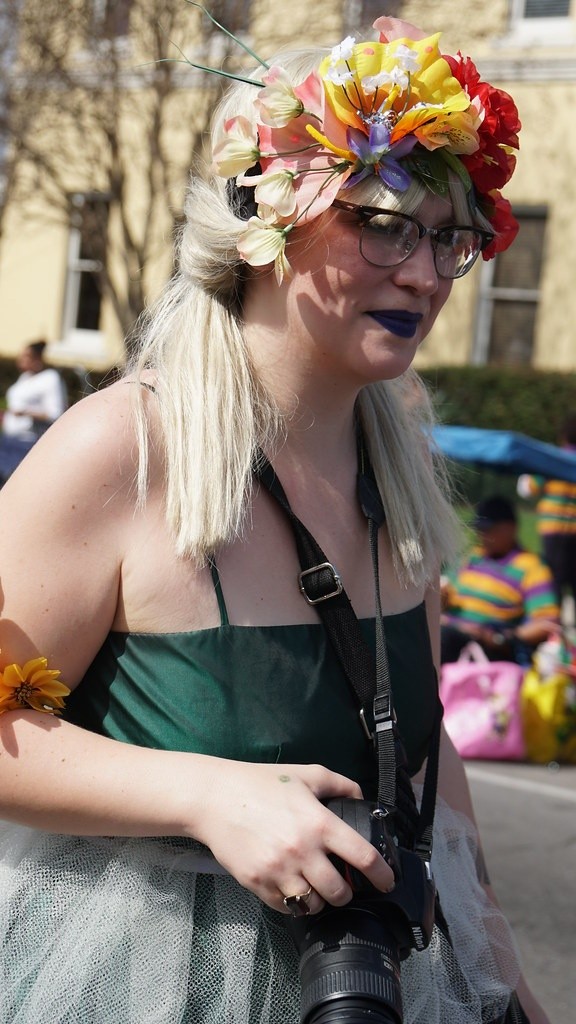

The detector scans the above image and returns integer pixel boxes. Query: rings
[284,888,312,919]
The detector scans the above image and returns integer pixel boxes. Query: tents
[418,421,576,482]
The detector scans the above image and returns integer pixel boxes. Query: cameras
[260,796,436,1024]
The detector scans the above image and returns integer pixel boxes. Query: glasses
[331,198,494,279]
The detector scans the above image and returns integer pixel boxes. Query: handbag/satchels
[438,641,528,761]
[520,668,576,765]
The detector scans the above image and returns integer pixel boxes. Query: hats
[462,493,514,530]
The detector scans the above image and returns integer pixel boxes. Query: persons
[439,494,558,667]
[518,433,576,601]
[2,340,69,441]
[0,47,552,1024]
